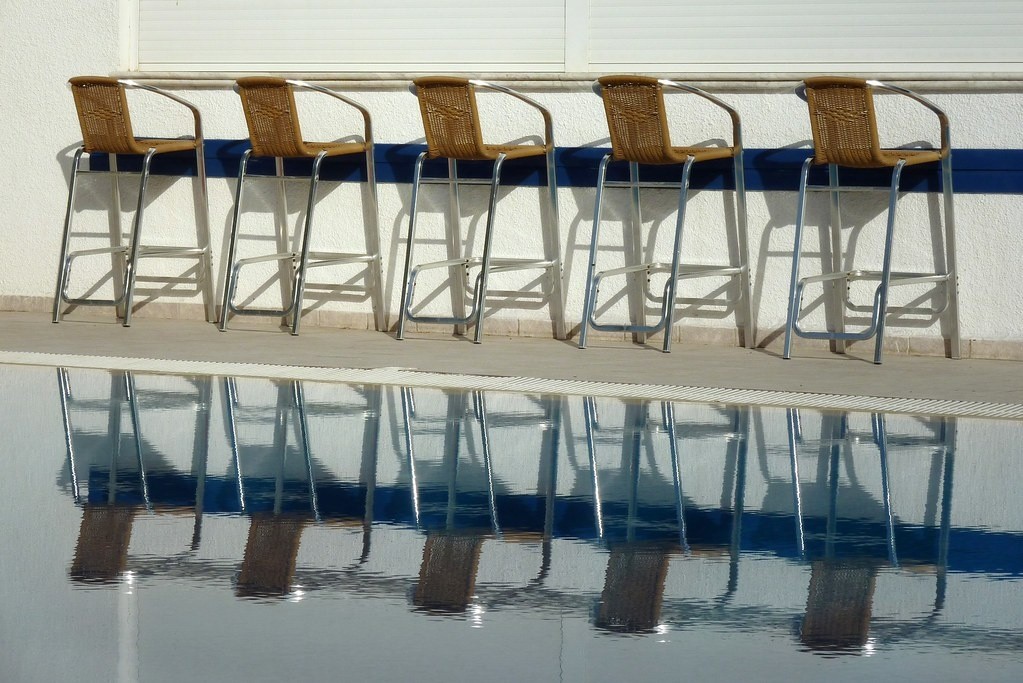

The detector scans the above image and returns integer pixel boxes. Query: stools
[54,368,962,658]
[395,73,568,341]
[783,75,962,364]
[221,69,385,336]
[579,76,754,351]
[50,76,215,326]
